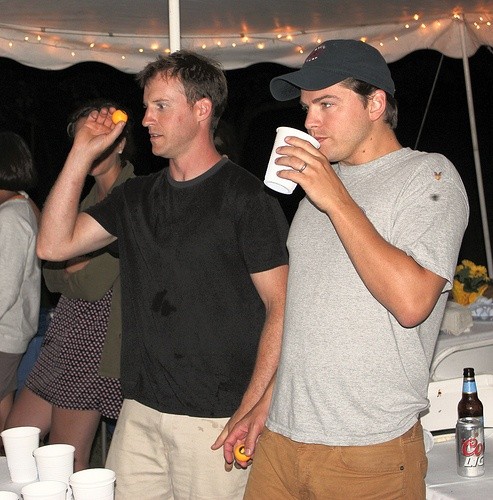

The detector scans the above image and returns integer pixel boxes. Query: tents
[1,0,492,277]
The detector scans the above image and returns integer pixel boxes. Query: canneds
[456,417,485,478]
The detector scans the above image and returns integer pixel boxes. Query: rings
[299,163,307,173]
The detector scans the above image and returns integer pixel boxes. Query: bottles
[458,367,485,454]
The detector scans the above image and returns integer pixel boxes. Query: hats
[270,38,395,102]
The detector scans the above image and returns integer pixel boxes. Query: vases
[451,280,485,306]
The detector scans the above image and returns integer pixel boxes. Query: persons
[5,100,137,471]
[37,50,291,499]
[224,39,468,500]
[0,129,41,439]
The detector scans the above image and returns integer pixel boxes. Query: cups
[262,125,322,194]
[68,466,118,500]
[32,444,76,500]
[20,480,68,500]
[0,490,19,500]
[0,426,41,483]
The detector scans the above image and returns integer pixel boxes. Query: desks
[422,430,493,500]
[15,306,117,469]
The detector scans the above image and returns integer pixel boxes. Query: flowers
[452,258,493,290]
